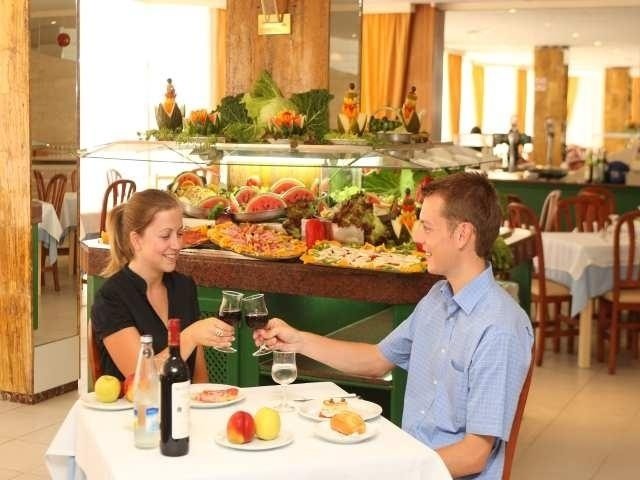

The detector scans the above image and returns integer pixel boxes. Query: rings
[214,328,225,337]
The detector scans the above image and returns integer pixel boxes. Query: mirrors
[330,1,363,133]
[27,0,78,348]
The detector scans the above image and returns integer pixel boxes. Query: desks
[78,229,540,430]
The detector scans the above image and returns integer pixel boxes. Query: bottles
[583,148,609,183]
[132,335,161,450]
[160,319,191,457]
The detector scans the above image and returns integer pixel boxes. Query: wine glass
[239,293,280,356]
[270,348,299,412]
[213,290,244,356]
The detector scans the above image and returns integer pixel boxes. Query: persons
[252,171,535,480]
[90,187,237,398]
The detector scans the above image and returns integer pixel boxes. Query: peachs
[226,411,257,445]
[122,373,136,401]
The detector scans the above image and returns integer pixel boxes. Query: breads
[331,410,367,436]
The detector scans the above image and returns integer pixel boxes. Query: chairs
[86,315,102,390]
[30,148,77,294]
[502,333,535,479]
[98,169,136,239]
[506,184,640,376]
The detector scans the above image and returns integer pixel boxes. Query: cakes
[319,397,349,418]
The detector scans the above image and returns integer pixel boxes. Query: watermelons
[171,171,327,217]
[267,115,308,137]
[369,118,402,133]
[182,110,223,138]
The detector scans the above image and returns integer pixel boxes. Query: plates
[528,168,572,178]
[315,423,378,444]
[183,193,329,223]
[301,397,383,423]
[80,392,133,412]
[268,132,430,146]
[192,137,226,143]
[214,431,292,453]
[190,383,246,408]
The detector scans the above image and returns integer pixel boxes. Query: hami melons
[335,113,368,136]
[154,102,186,131]
[397,107,427,134]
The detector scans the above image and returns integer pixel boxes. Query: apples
[255,406,281,441]
[94,375,121,403]
